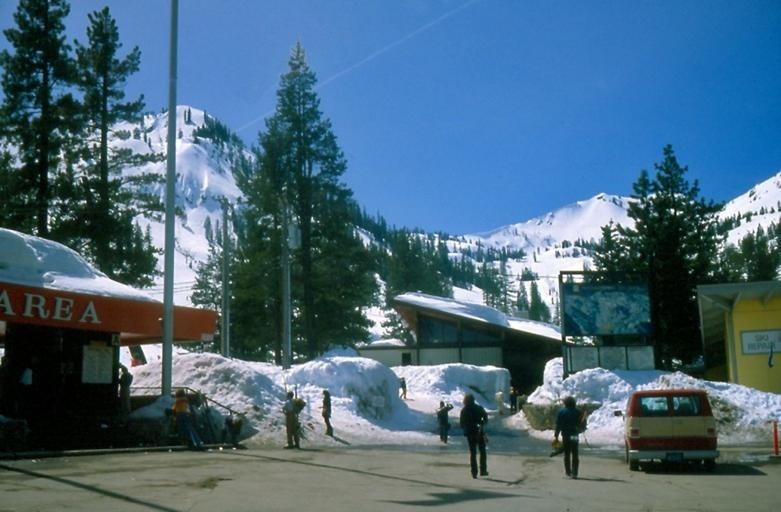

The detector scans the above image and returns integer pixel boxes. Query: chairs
[677,402,692,415]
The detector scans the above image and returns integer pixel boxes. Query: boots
[325,428,332,436]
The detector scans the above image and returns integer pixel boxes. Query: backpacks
[439,411,448,424]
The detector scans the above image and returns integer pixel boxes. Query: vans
[614,389,720,472]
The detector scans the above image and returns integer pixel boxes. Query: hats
[564,396,575,407]
[175,389,185,397]
[463,395,474,406]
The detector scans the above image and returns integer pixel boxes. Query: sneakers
[472,469,489,479]
[566,467,577,479]
[440,438,447,443]
[284,444,300,448]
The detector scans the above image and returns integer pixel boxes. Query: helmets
[551,439,562,449]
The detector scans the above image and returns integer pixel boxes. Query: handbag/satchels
[463,421,477,437]
[480,432,489,444]
[293,398,306,414]
[550,441,564,457]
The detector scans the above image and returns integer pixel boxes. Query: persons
[555,397,586,477]
[437,401,454,442]
[120,367,134,412]
[401,377,408,399]
[496,389,507,415]
[283,391,302,448]
[319,390,334,436]
[173,388,186,420]
[509,386,518,414]
[460,394,490,479]
